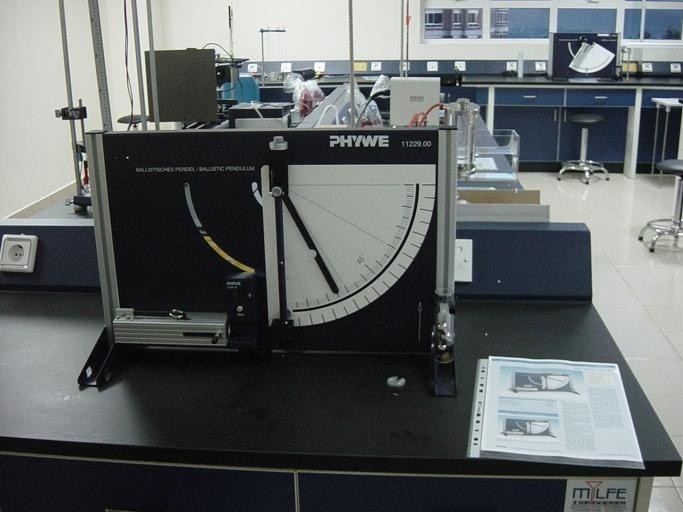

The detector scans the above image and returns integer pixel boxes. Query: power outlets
[0,234,38,274]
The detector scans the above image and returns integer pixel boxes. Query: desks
[458,113,524,189]
[651,97,683,176]
[0,290,683,511]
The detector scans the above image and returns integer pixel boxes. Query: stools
[558,114,610,184]
[638,160,683,252]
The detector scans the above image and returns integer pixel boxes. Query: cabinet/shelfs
[461,84,683,179]
